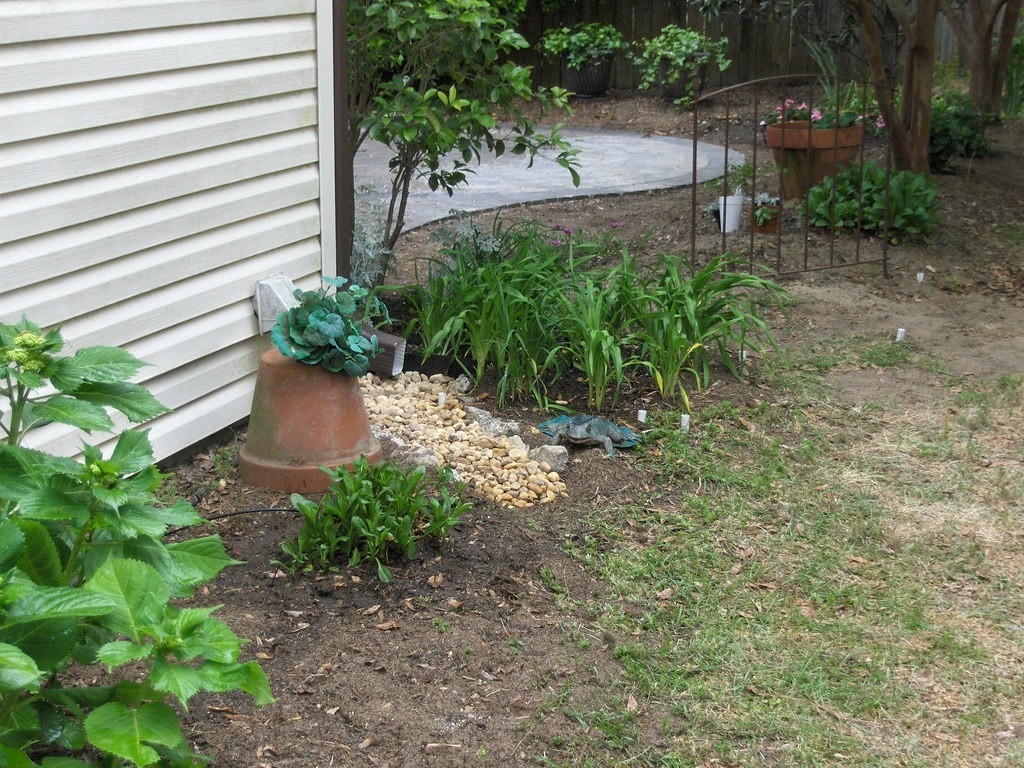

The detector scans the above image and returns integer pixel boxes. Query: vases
[239,334,385,498]
[752,206,781,233]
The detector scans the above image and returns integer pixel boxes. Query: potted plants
[699,158,791,232]
[632,23,733,114]
[532,21,630,99]
[766,29,865,199]
